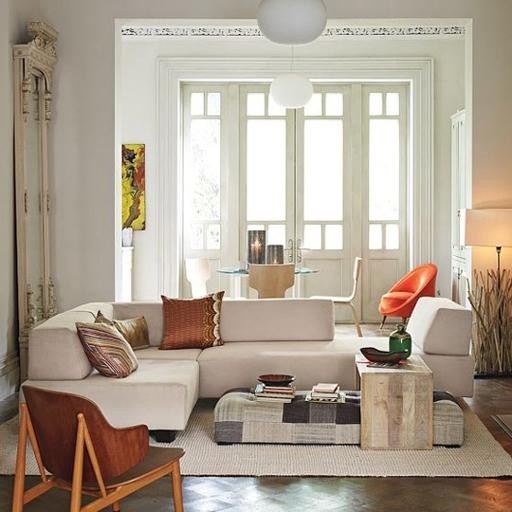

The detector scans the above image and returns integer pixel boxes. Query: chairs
[11,384,185,512]
[310,256,362,337]
[184,257,210,298]
[378,263,438,330]
[248,263,295,298]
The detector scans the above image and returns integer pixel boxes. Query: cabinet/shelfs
[448,108,467,307]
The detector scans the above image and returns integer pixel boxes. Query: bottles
[389,326,412,358]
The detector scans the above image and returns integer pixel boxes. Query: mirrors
[10,19,59,384]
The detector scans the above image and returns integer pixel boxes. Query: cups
[265,244,284,264]
[122,226,133,246]
[247,230,266,267]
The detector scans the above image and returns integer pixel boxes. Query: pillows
[158,290,226,350]
[74,310,138,378]
[112,316,151,351]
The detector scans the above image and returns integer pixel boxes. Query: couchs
[16,296,474,443]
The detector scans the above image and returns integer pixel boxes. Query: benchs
[213,387,464,448]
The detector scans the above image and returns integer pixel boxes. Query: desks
[217,263,320,275]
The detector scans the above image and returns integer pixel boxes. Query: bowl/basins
[256,374,295,385]
[360,346,408,363]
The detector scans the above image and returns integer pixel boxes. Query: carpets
[490,414,512,440]
[0,393,512,478]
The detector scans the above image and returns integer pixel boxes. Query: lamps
[462,207,512,379]
[269,44,314,108]
[256,0,328,44]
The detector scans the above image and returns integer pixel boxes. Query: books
[252,384,295,403]
[304,382,348,404]
